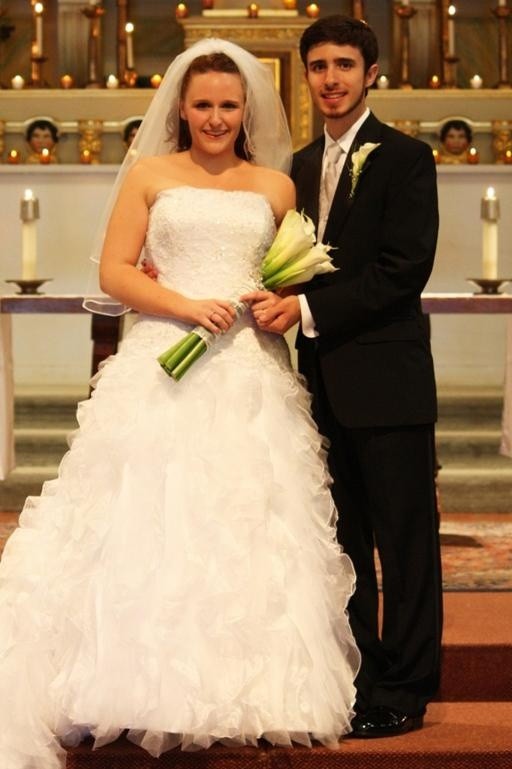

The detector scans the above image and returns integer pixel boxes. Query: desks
[0,286,512,396]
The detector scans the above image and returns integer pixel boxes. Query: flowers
[153,207,342,382]
[349,138,382,200]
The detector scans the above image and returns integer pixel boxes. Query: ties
[317,143,342,245]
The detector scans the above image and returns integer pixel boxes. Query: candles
[1,0,512,176]
[478,184,504,280]
[18,180,44,280]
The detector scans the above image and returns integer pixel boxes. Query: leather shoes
[352,705,427,738]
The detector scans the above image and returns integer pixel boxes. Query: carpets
[1,508,512,597]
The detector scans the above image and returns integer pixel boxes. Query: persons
[1,36,361,768]
[26,119,58,163]
[123,119,142,147]
[439,119,472,155]
[255,14,442,739]
